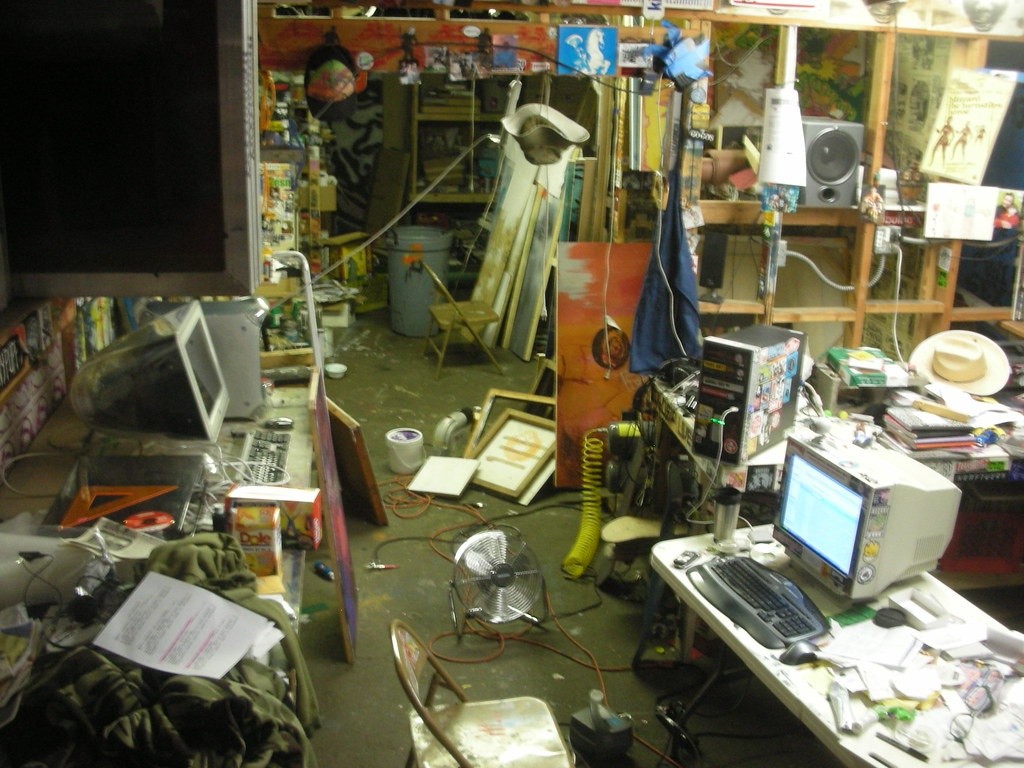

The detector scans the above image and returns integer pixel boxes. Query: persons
[995,192,1020,229]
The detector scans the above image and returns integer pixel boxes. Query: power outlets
[777,240,787,267]
[873,225,902,254]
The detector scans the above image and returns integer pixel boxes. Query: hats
[910,330,1011,397]
[499,103,590,165]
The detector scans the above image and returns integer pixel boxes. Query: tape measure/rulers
[54,485,179,530]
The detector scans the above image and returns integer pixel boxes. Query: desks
[651,524,1024,768]
[651,377,1024,662]
[0,381,313,637]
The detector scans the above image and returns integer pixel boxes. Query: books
[883,407,979,460]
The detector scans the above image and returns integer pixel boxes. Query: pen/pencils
[877,730,929,765]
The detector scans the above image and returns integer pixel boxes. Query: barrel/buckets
[388,224,453,336]
[386,427,427,473]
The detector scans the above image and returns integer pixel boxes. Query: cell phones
[673,550,702,569]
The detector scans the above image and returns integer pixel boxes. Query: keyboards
[236,429,291,486]
[686,556,831,649]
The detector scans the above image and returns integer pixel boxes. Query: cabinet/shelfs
[409,72,506,201]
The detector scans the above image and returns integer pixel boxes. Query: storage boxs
[329,242,372,281]
[222,485,325,576]
[301,185,337,211]
[828,345,909,390]
[692,325,810,464]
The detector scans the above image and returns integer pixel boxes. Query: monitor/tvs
[771,434,962,608]
[70,299,230,443]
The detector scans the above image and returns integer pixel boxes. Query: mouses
[779,639,822,665]
[265,418,294,430]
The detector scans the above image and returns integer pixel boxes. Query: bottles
[713,486,742,542]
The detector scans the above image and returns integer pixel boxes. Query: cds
[125,511,174,533]
[750,543,790,571]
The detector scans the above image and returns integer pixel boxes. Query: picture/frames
[467,408,558,498]
[466,359,556,457]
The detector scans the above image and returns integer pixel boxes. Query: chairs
[389,619,575,768]
[421,261,506,376]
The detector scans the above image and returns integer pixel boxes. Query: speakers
[696,232,728,303]
[796,115,864,207]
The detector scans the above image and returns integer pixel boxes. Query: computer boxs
[134,296,270,421]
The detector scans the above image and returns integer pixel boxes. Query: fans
[449,530,556,641]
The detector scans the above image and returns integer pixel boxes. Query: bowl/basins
[326,363,347,379]
